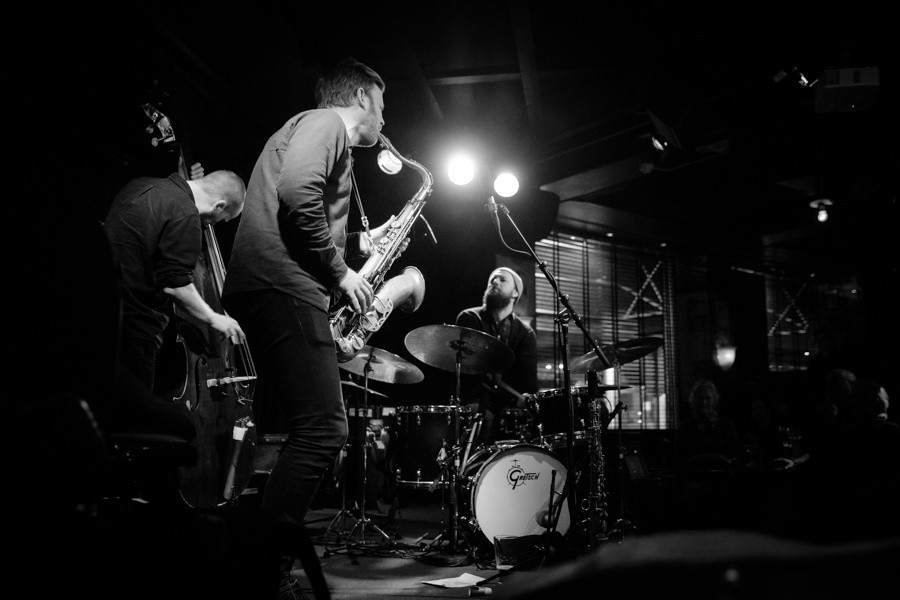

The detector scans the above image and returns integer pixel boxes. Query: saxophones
[330,131,435,363]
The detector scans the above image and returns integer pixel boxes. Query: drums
[527,389,587,449]
[497,408,527,436]
[456,445,571,549]
[365,402,470,490]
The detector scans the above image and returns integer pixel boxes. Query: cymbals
[340,381,389,401]
[566,335,666,373]
[574,386,633,391]
[403,325,515,377]
[339,344,425,386]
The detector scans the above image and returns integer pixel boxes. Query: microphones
[487,195,500,231]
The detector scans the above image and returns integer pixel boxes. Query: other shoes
[278,574,308,600]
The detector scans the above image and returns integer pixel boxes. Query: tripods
[318,379,403,549]
[421,352,478,558]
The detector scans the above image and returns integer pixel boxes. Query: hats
[491,267,524,306]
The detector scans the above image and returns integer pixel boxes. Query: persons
[456,266,539,426]
[682,379,728,467]
[217,59,387,599]
[96,157,247,513]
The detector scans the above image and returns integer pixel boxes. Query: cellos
[135,102,258,511]
